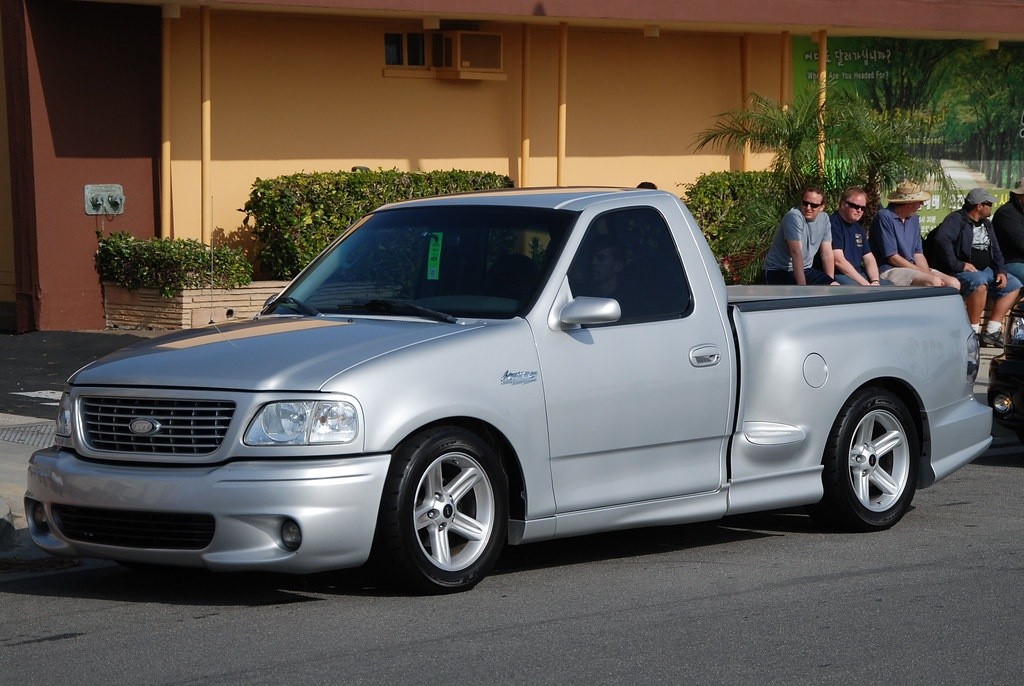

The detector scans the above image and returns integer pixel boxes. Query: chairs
[483,254,538,295]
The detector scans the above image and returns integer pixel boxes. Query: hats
[1010,176,1024,198]
[886,179,931,204]
[965,188,999,205]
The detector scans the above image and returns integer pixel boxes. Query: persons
[870,178,961,290]
[992,177,1024,295]
[763,184,840,286]
[576,237,655,320]
[933,188,1024,347]
[638,182,657,189]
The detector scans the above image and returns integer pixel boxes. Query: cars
[987,300,1024,437]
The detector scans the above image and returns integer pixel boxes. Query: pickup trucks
[22,187,994,596]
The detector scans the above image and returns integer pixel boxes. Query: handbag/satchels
[922,210,967,276]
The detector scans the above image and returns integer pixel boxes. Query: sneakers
[975,329,1004,348]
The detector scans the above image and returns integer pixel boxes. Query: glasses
[984,202,992,207]
[801,197,822,208]
[846,201,867,211]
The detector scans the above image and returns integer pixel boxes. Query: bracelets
[813,187,894,286]
[870,280,880,283]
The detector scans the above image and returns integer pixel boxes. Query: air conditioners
[443,30,504,72]
